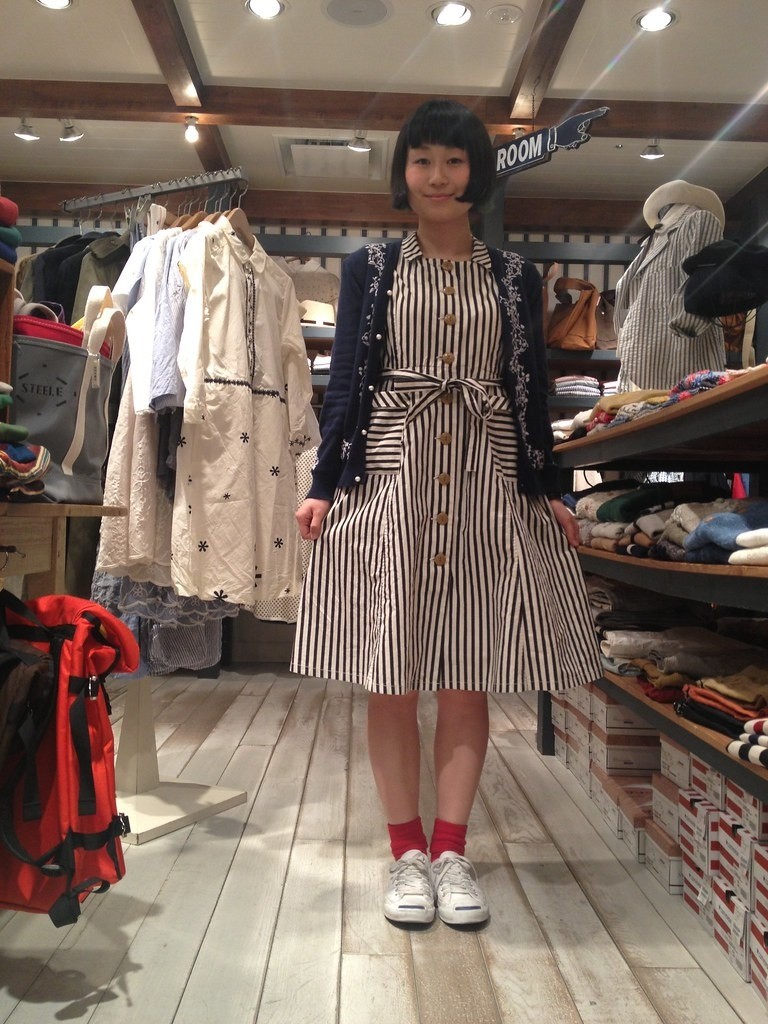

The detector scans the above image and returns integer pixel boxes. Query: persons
[286,99,609,926]
[606,179,729,394]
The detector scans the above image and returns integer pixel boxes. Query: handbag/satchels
[11,305,127,503]
[15,284,113,357]
[547,276,600,350]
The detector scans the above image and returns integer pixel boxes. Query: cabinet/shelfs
[536,362,768,807]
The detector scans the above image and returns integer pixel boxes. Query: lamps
[14,117,39,141]
[348,130,371,152]
[640,138,664,160]
[59,119,84,141]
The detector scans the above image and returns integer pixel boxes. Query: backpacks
[0,596,138,928]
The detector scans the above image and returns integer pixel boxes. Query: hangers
[49,165,255,251]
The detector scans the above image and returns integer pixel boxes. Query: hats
[643,180,725,233]
[681,240,768,317]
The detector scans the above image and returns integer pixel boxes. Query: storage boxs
[548,682,768,1016]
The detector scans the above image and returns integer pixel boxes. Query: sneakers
[430,849,490,925]
[383,848,435,924]
